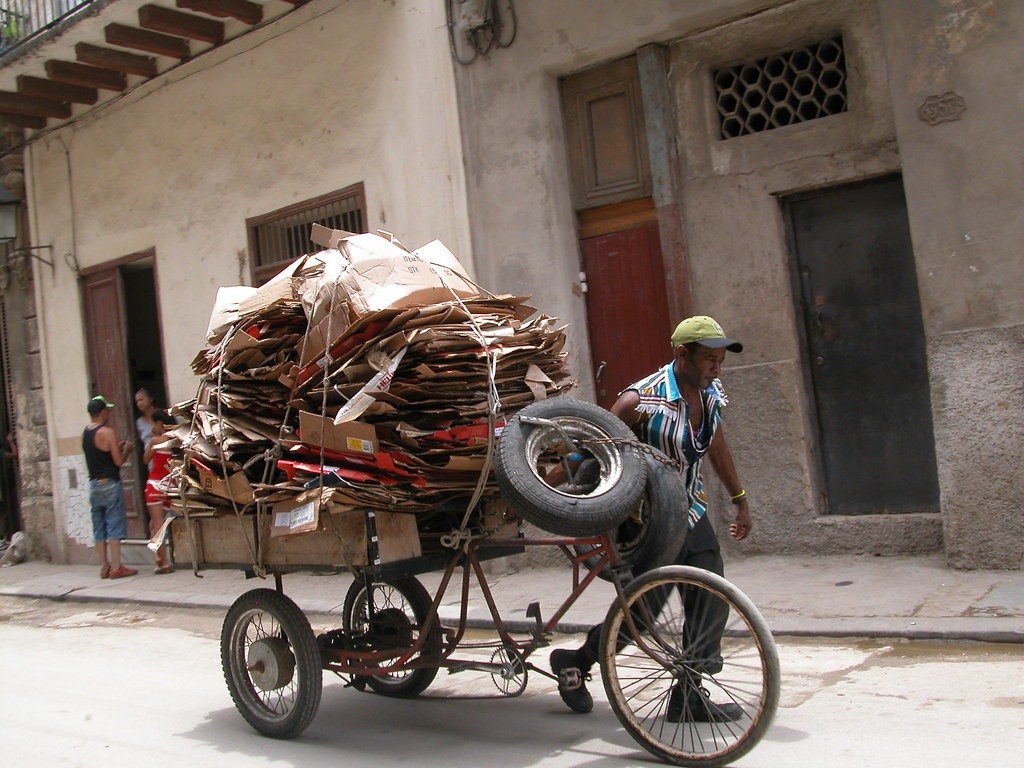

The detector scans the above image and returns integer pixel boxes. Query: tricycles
[218,413,782,768]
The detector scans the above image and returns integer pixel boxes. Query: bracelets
[730,489,748,504]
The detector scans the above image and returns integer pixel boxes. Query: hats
[670,316,743,353]
[88,395,115,412]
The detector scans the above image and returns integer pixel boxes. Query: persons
[133,386,178,575]
[0,432,25,567]
[504,315,753,723]
[80,395,138,581]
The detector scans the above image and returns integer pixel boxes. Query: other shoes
[101,564,110,579]
[154,562,173,574]
[550,648,593,713]
[667,684,743,722]
[110,564,138,579]
